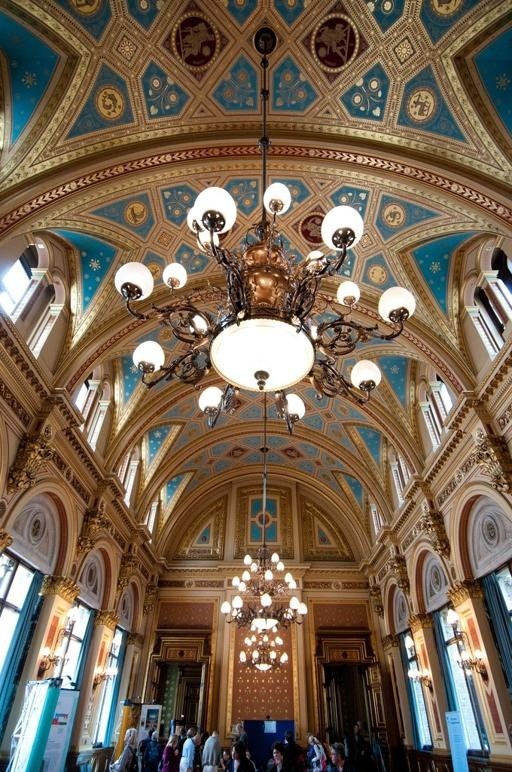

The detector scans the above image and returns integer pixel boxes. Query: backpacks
[125,746,140,771]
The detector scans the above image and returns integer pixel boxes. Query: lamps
[113,25,417,428]
[40,604,118,689]
[220,449,306,667]
[401,610,490,690]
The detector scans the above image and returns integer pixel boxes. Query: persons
[299,216,322,241]
[182,26,216,61]
[108,722,387,772]
[314,24,348,64]
